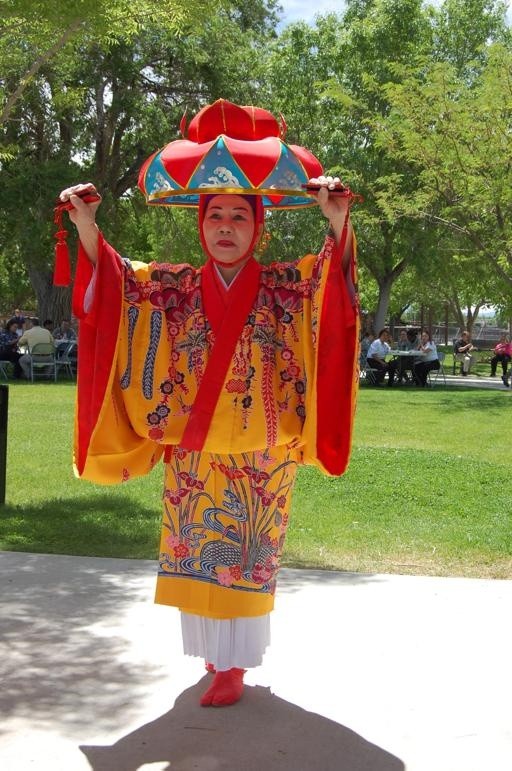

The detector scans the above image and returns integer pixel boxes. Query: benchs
[453,346,512,376]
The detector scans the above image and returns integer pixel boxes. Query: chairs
[359,346,448,388]
[0,333,77,384]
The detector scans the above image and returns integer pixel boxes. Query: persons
[359,328,440,388]
[58,174,361,710]
[489,335,510,378]
[1,308,76,381]
[501,339,511,387]
[452,329,479,375]
